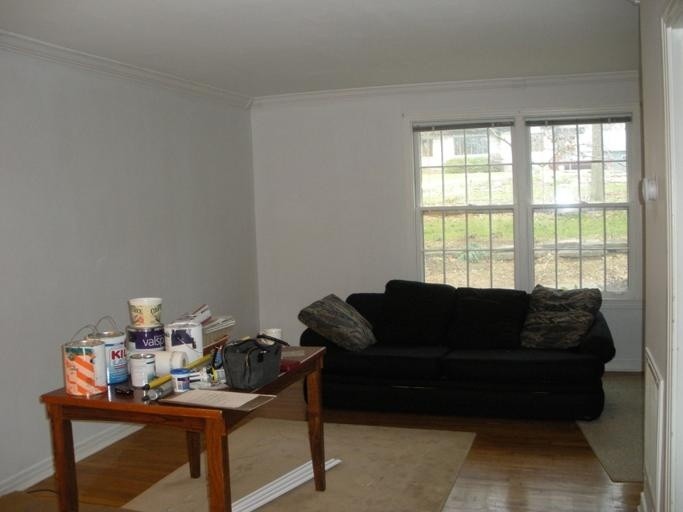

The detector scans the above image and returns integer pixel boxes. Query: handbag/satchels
[223,338,281,391]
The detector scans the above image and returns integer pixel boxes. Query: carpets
[121,417,477,512]
[575,374,643,483]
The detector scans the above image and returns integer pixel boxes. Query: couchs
[300,293,615,421]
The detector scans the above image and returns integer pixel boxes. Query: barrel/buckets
[62,324,107,398]
[165,322,203,361]
[87,316,129,383]
[127,327,164,375]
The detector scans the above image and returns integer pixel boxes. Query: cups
[260,327,283,348]
[170,368,191,394]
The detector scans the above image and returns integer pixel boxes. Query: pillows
[385,280,602,350]
[298,294,377,351]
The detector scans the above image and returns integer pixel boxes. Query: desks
[40,347,327,512]
